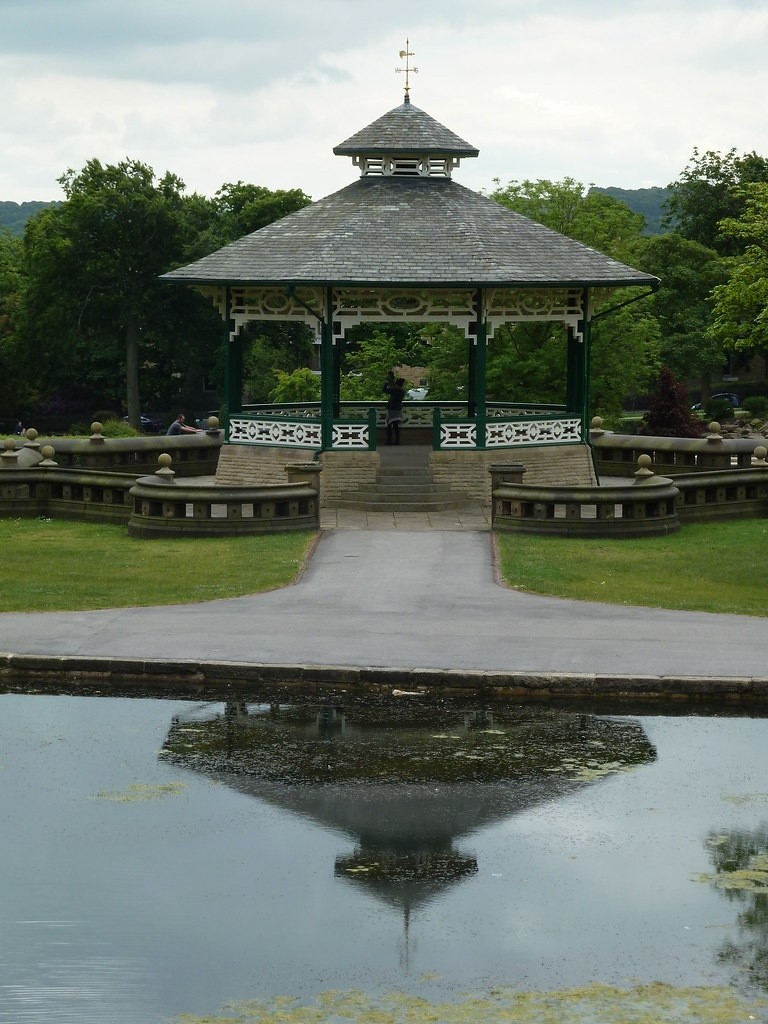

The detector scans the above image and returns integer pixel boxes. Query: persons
[383,370,405,446]
[15,421,23,435]
[166,414,201,435]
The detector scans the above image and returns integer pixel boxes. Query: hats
[395,378,405,387]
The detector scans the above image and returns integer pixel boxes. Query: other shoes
[384,440,391,445]
[393,440,400,445]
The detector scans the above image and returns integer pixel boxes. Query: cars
[120,414,159,434]
[692,393,744,412]
